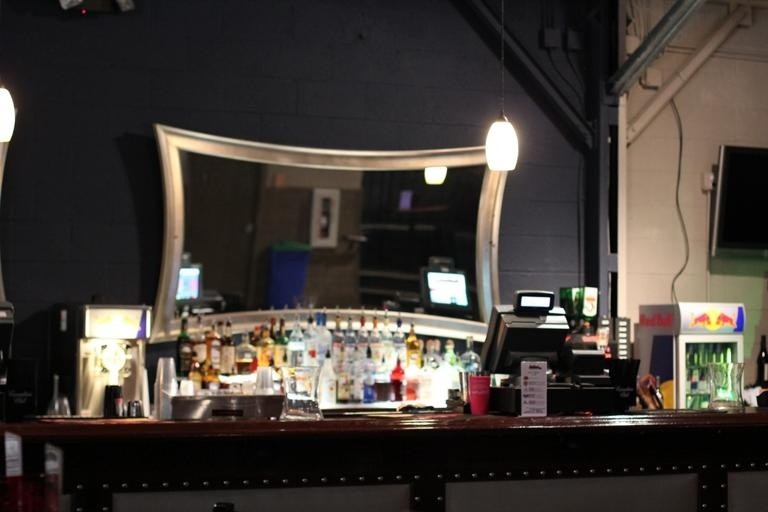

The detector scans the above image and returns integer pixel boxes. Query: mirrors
[149,123,511,345]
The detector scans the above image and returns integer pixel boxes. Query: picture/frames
[310,186,340,250]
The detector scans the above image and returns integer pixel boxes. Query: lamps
[1,87,17,383]
[485,0,519,171]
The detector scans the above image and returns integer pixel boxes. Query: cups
[47,395,72,417]
[154,358,179,420]
[279,365,325,422]
[468,375,491,415]
[127,399,144,417]
[103,384,125,418]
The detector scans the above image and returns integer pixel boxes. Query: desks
[0,398,768,512]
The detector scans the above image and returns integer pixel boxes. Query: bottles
[177,302,482,404]
[755,335,768,389]
[686,343,738,409]
[558,285,600,327]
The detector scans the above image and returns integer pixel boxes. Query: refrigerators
[632,301,746,411]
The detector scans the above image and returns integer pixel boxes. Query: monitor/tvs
[176,264,204,302]
[420,268,473,313]
[475,304,570,375]
[710,145,768,257]
[171,395,285,420]
